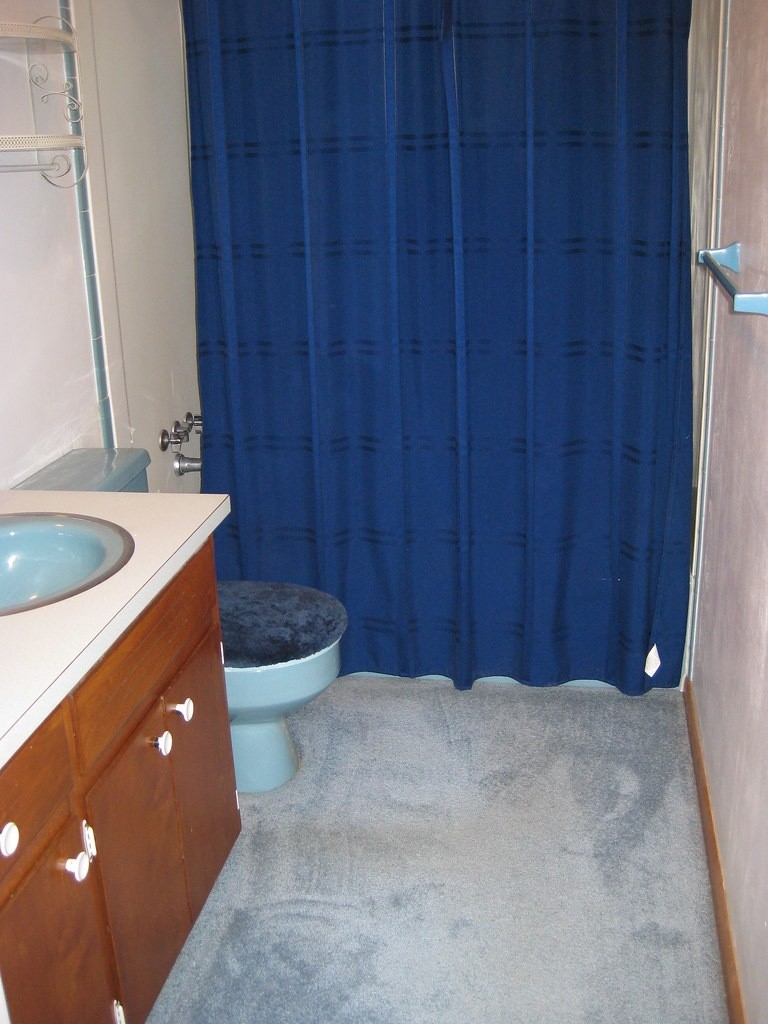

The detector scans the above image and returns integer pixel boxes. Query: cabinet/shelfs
[68,531,242,1024]
[0,695,116,1024]
[0,16,89,188]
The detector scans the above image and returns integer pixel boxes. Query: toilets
[10,445,351,793]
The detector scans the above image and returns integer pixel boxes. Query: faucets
[172,452,202,475]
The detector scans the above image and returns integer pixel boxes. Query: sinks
[0,510,136,617]
